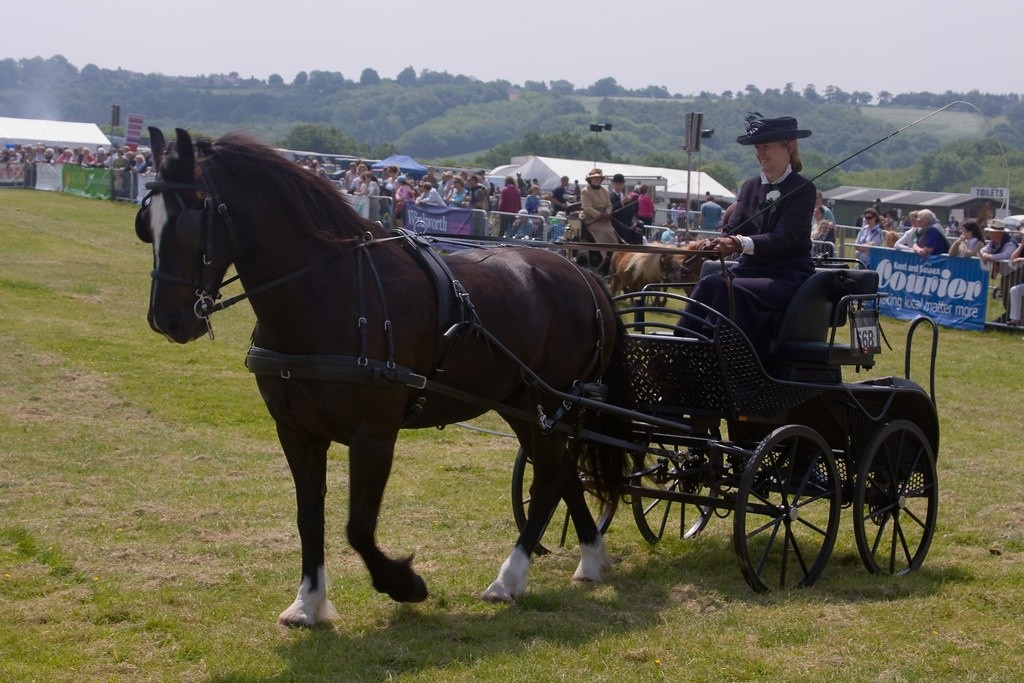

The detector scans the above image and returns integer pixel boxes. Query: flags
[125,116,143,152]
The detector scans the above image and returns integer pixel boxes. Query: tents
[507,155,737,211]
[371,154,428,181]
[822,185,1013,238]
[0,117,112,155]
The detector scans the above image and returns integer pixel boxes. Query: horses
[607,238,712,308]
[134,124,677,631]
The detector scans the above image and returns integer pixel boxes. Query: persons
[673,117,816,411]
[811,190,836,266]
[344,162,655,246]
[853,206,1024,325]
[296,156,328,178]
[0,142,154,186]
[654,191,724,245]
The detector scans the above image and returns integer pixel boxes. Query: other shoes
[1006,320,1022,326]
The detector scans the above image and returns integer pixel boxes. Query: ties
[618,193,621,199]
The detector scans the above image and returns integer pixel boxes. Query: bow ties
[762,182,783,193]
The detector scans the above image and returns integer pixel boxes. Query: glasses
[865,215,875,219]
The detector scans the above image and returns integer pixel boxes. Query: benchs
[699,260,880,365]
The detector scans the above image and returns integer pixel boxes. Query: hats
[515,208,529,218]
[548,210,568,221]
[586,169,608,179]
[984,219,1010,233]
[394,175,407,184]
[1016,224,1024,234]
[736,113,812,145]
[610,175,626,182]
[538,206,550,213]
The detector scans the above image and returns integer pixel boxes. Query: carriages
[558,220,740,310]
[131,119,941,627]
